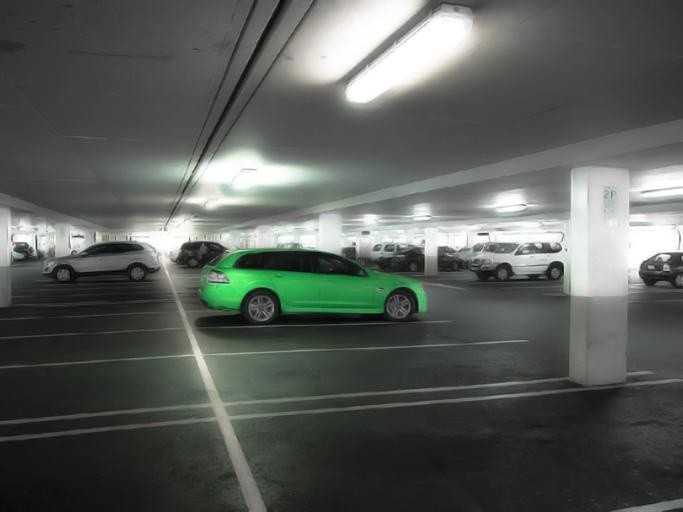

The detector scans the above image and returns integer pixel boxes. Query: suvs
[470,240,568,281]
[39,239,160,284]
[177,241,227,268]
[369,242,415,266]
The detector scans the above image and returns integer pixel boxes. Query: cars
[454,246,471,258]
[35,244,80,258]
[376,245,467,271]
[164,244,180,265]
[343,248,356,259]
[638,252,683,288]
[9,241,30,262]
[276,241,302,249]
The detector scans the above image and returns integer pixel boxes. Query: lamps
[345,4,474,103]
[15,164,683,244]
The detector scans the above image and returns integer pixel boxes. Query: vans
[196,246,426,324]
